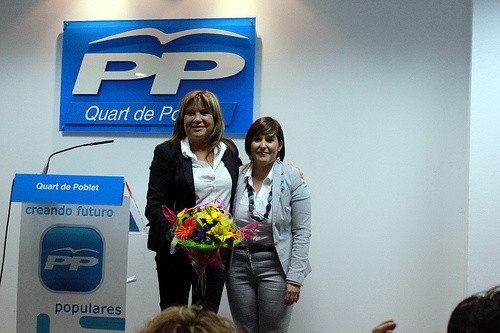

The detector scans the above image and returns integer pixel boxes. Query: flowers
[159,203,260,297]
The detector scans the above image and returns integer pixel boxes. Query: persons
[225,117,311,333]
[138,306,241,333]
[372,285,500,333]
[145,91,304,315]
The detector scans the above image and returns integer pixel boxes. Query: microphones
[45,140,114,175]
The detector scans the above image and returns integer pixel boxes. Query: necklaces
[246,177,272,222]
